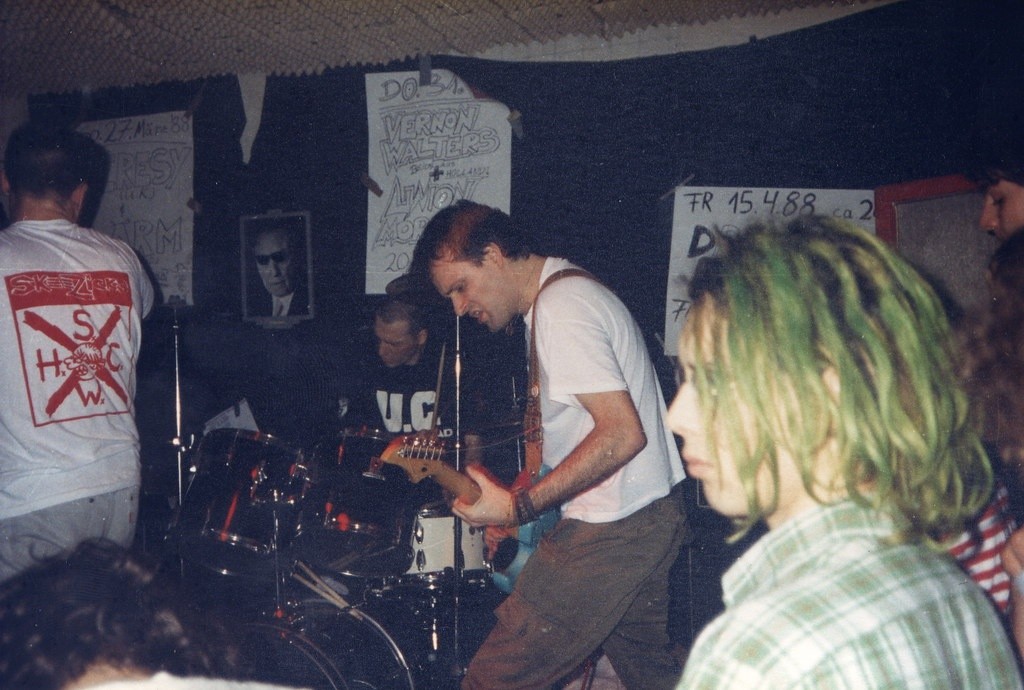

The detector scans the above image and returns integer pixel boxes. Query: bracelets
[499,488,538,530]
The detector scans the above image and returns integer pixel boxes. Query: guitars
[382,427,531,570]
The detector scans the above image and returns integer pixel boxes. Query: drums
[305,425,402,550]
[172,429,309,584]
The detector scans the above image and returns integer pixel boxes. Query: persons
[942,117,1024,662]
[410,198,697,690]
[0,541,253,690]
[666,213,1024,690]
[253,224,308,315]
[0,122,155,585]
[370,301,489,467]
